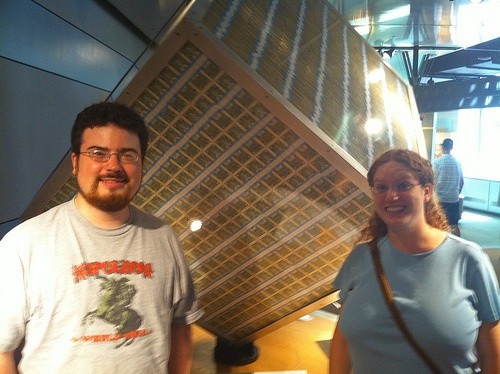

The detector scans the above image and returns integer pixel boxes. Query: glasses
[76,149,145,163]
[369,182,425,194]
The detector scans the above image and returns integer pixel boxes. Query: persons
[432,138,464,237]
[329,148,500,374]
[0,103,195,374]
